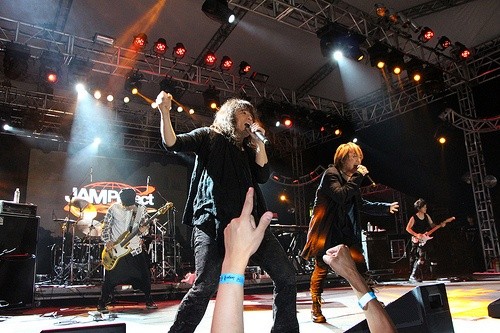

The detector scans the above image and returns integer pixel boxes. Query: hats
[120,189,136,206]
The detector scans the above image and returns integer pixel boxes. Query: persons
[465,215,478,243]
[310,142,399,323]
[156,90,300,333]
[406,198,446,284]
[97,188,159,313]
[322,244,398,333]
[211,187,274,333]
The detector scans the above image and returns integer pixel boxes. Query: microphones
[357,165,376,186]
[147,176,149,191]
[248,125,268,144]
[90,168,92,182]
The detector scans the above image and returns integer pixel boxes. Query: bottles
[13,188,20,203]
[367,222,371,231]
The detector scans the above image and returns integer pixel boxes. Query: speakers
[0,255,37,308]
[0,214,40,255]
[342,283,454,333]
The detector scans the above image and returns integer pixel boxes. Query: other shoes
[410,275,422,282]
[146,301,158,308]
[98,304,109,313]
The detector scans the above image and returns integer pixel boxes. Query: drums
[73,244,93,269]
[93,243,104,265]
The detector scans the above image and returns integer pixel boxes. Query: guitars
[411,217,455,246]
[101,201,173,270]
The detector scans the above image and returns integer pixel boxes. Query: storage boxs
[245,266,261,281]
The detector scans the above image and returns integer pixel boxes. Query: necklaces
[340,168,352,177]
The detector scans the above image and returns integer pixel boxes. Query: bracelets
[219,272,245,286]
[358,291,377,309]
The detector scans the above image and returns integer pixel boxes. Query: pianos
[270,224,309,276]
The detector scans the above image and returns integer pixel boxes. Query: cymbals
[77,220,102,236]
[53,218,76,223]
[70,199,97,220]
[147,208,157,213]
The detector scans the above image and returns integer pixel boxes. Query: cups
[370,226,373,231]
[375,226,378,231]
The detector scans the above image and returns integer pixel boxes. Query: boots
[368,284,385,309]
[310,289,327,323]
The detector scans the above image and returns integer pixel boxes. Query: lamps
[158,76,178,104]
[131,32,149,53]
[40,51,64,83]
[315,2,471,82]
[87,75,102,99]
[150,37,168,58]
[172,43,186,64]
[308,110,329,131]
[202,50,217,70]
[279,102,293,129]
[432,119,452,145]
[220,55,233,74]
[124,68,144,95]
[3,40,32,82]
[203,84,221,111]
[328,115,343,138]
[345,130,358,143]
[257,100,281,128]
[199,0,236,25]
[238,61,251,78]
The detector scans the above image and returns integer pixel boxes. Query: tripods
[55,173,92,286]
[146,213,178,280]
